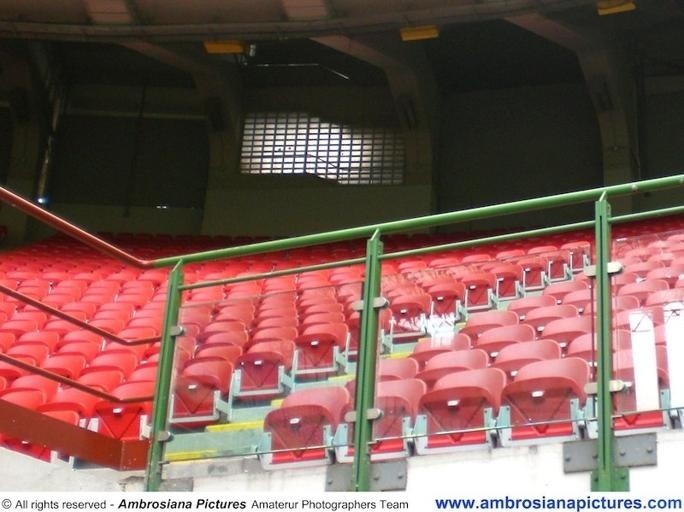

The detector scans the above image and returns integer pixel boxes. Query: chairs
[0,208,684,478]
